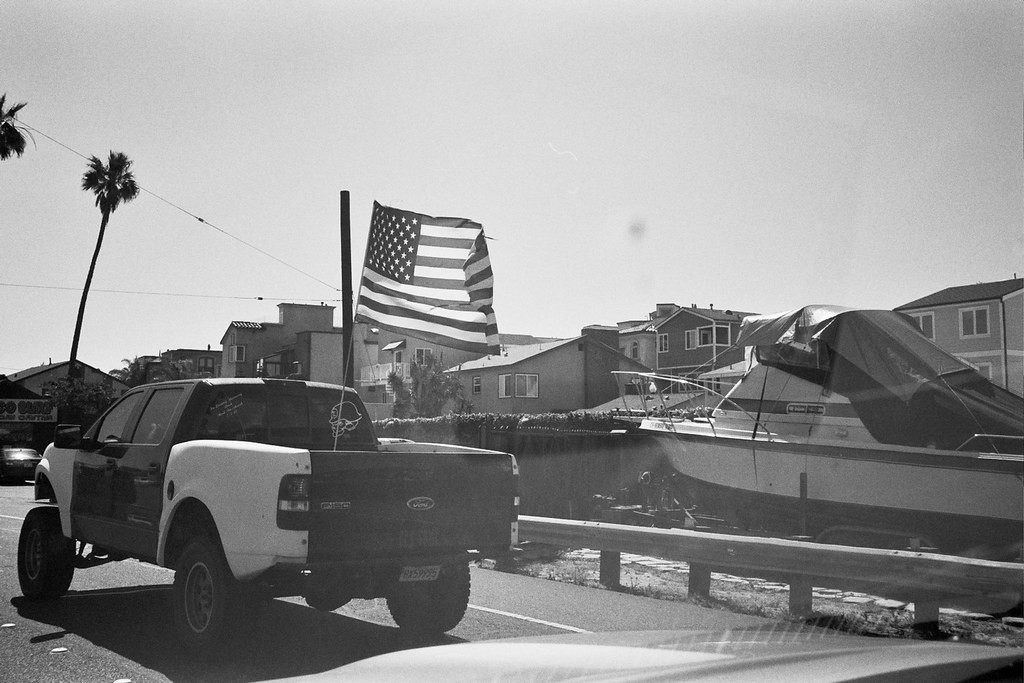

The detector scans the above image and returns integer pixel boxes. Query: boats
[608,305,1024,567]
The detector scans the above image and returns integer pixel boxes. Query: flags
[353,199,502,357]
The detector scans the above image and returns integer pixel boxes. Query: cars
[0,448,42,484]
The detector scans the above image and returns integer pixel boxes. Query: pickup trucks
[16,376,526,656]
